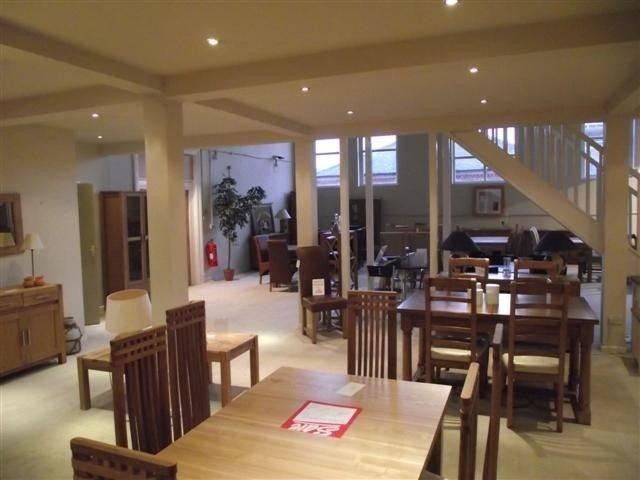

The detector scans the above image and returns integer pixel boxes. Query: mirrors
[1,193,25,256]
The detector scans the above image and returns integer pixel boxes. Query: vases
[66,316,82,355]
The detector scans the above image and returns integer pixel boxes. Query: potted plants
[212,177,264,281]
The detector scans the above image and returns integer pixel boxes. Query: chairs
[70,437,178,479]
[421,230,596,432]
[257,228,421,290]
[109,326,171,455]
[297,246,347,341]
[484,323,504,478]
[164,299,212,432]
[344,288,397,379]
[422,361,483,480]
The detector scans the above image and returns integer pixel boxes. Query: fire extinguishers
[207,238,217,266]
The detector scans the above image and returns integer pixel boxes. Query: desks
[398,285,596,430]
[153,367,452,480]
[77,325,260,411]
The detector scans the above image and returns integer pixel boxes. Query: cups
[503,257,511,274]
[486,284,501,305]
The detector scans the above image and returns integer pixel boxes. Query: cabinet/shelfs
[249,232,287,273]
[0,280,66,379]
[103,190,150,303]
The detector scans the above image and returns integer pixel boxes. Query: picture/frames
[248,202,276,235]
[474,186,506,218]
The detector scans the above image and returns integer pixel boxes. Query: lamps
[20,233,47,286]
[105,289,153,335]
[276,209,292,232]
[1,232,17,248]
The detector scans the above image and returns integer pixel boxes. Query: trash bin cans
[367,265,391,291]
